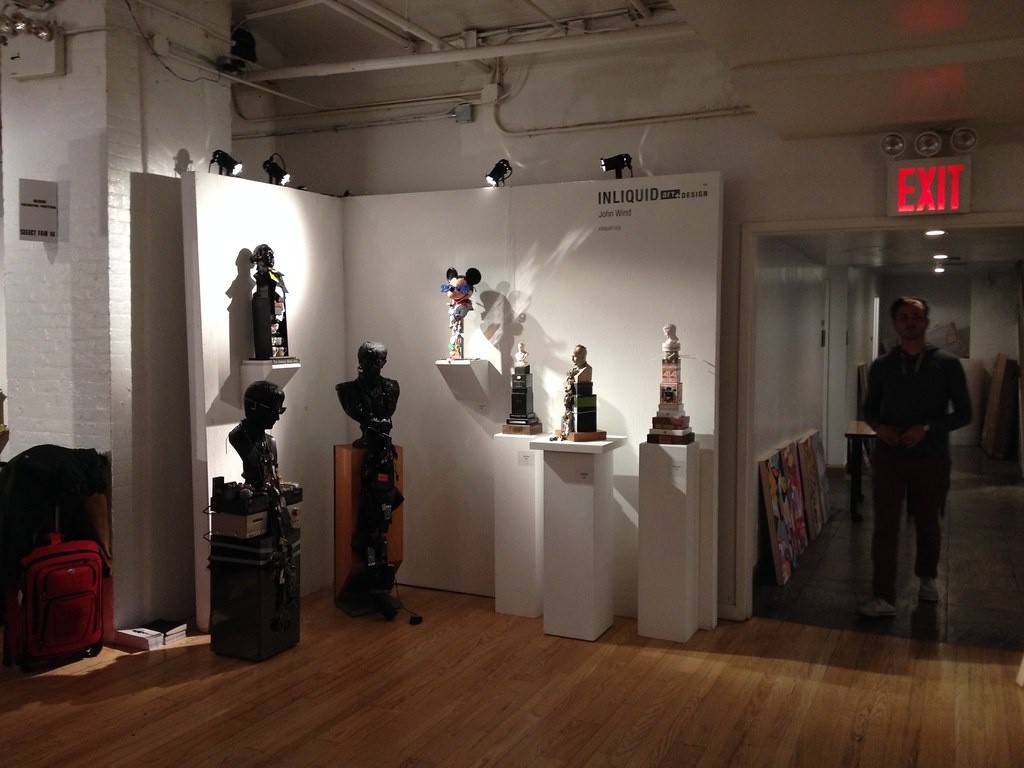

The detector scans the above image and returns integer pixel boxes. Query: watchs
[921,422,932,437]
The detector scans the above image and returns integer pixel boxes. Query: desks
[845,420,879,521]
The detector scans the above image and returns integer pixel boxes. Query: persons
[855,296,973,616]
[572,344,592,382]
[250,244,279,278]
[663,323,680,351]
[335,340,400,451]
[515,343,530,366]
[228,380,287,492]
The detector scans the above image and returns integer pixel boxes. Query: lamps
[600,154,634,179]
[262,156,290,185]
[208,149,243,176]
[880,127,977,216]
[485,158,513,187]
[0,11,66,78]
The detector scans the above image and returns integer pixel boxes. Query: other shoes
[918,578,939,601]
[862,597,896,614]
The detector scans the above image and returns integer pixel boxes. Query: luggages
[3,501,104,675]
